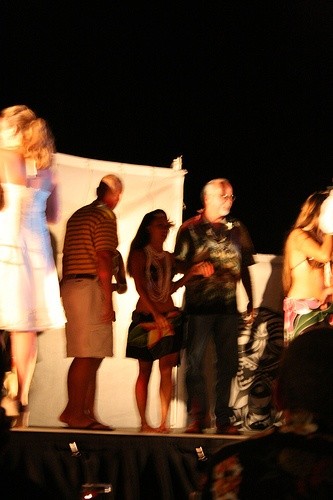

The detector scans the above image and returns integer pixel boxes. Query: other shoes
[216,426,243,435]
[184,422,204,434]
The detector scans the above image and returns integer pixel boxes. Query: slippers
[62,422,113,431]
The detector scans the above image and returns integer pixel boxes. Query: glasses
[220,194,235,201]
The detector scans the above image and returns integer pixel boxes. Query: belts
[64,273,97,279]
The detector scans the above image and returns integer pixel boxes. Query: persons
[199,328,333,500]
[125,209,203,434]
[59,174,128,432]
[0,105,68,428]
[281,189,333,342]
[174,178,255,435]
